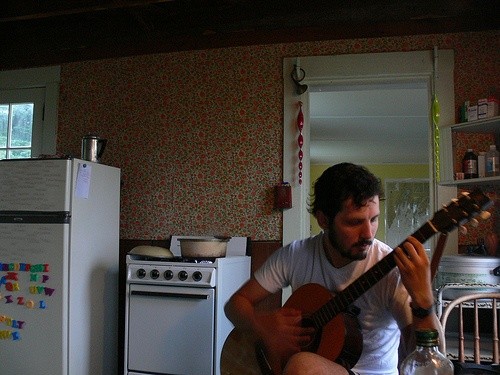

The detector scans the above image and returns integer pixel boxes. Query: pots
[434,243,500,299]
[177,238,231,259]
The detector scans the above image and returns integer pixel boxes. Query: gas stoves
[125,234,252,267]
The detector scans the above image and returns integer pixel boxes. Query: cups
[81,130,108,164]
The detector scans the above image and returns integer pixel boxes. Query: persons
[223,162,446,375]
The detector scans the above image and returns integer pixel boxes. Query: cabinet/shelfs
[440,115,500,187]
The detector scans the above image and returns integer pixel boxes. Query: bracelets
[410,302,436,318]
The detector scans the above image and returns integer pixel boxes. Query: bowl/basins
[127,246,174,258]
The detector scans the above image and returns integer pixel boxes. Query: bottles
[463,149,478,179]
[399,328,454,375]
[486,145,500,177]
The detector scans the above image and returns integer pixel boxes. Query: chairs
[440,292,500,375]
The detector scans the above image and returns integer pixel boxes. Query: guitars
[219,187,495,375]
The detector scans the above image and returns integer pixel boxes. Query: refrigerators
[0,157,122,374]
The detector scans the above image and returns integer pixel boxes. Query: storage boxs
[460,96,499,123]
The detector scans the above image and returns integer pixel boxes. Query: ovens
[123,264,250,374]
